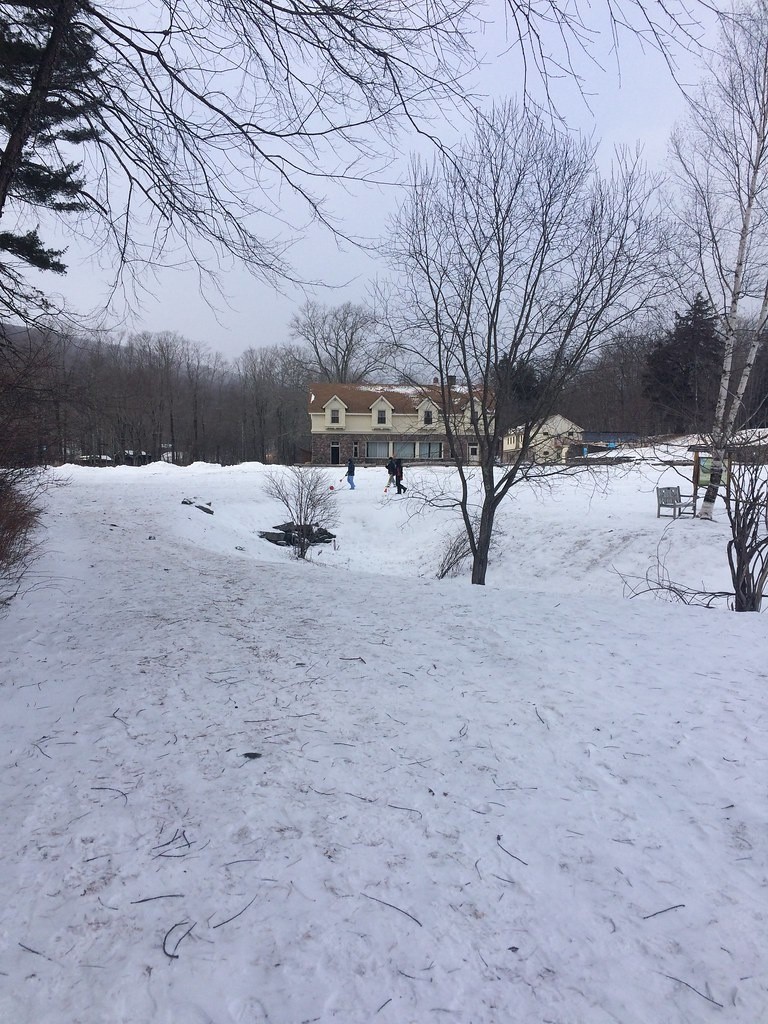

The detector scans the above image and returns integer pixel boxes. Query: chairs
[657,485,697,520]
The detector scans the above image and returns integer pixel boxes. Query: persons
[395,459,407,494]
[346,458,355,491]
[384,457,395,493]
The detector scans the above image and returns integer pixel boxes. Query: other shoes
[403,488,407,493]
[385,486,389,487]
[350,488,354,490]
[394,485,396,487]
[396,493,401,494]
